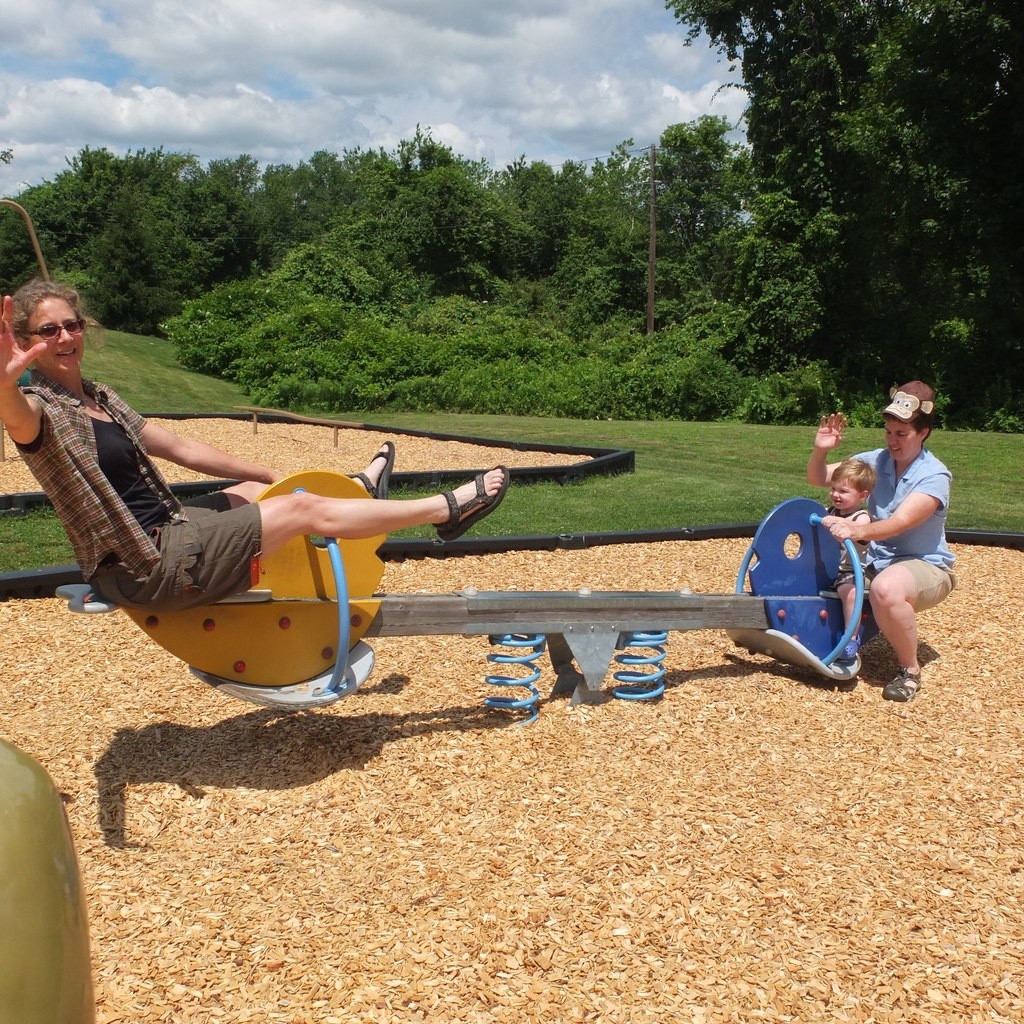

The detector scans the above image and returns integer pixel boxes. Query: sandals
[349,441,395,500]
[431,465,509,541]
[882,665,922,701]
[836,633,861,659]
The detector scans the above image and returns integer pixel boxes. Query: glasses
[25,319,85,341]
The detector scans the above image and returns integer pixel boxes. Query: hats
[883,381,935,424]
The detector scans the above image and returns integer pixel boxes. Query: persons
[0,280,509,609]
[827,460,876,660]
[806,381,957,702]
